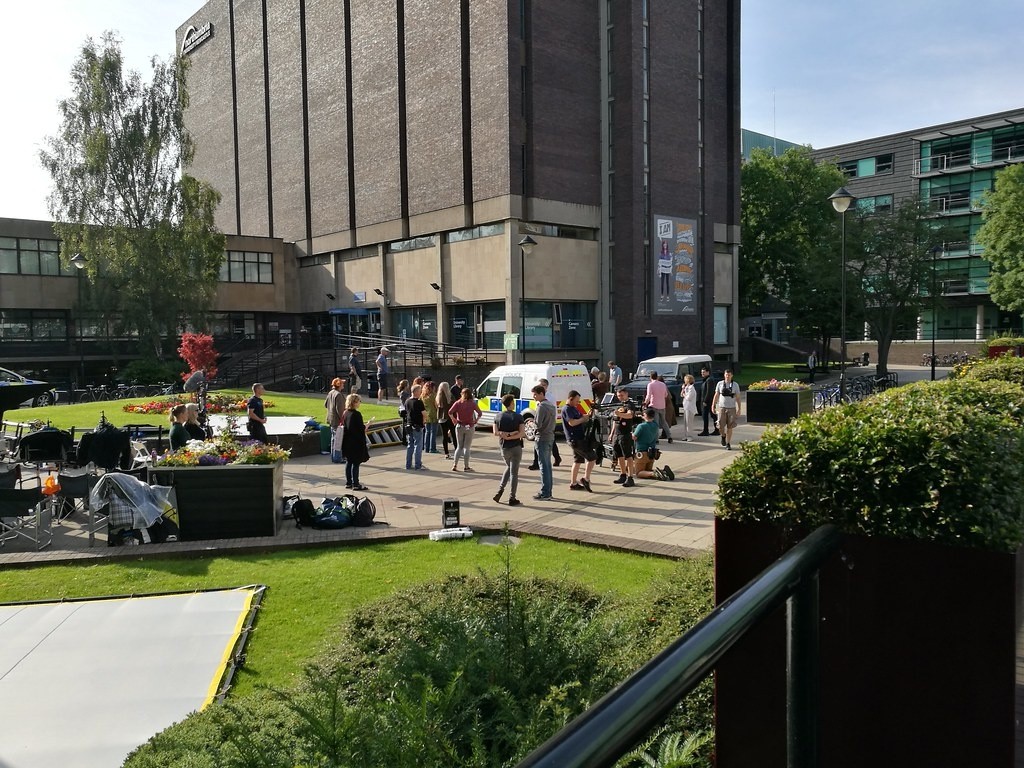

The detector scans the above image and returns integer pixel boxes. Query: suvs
[618,353,714,420]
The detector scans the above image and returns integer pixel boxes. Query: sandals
[353,485,368,490]
[345,483,353,489]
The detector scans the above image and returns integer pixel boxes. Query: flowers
[154,412,293,467]
[747,377,813,391]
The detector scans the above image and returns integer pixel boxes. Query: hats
[381,347,389,351]
[331,378,346,387]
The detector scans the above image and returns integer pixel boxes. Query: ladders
[365,424,404,448]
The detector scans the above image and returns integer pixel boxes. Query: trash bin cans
[367,373,378,398]
[862,352,869,366]
[320,426,331,454]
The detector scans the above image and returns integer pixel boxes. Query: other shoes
[553,458,562,466]
[580,478,592,493]
[664,465,674,480]
[613,474,627,484]
[493,490,504,503]
[533,492,552,501]
[652,467,664,481]
[509,499,520,506]
[424,447,454,460]
[333,458,345,463]
[528,464,539,470]
[659,430,731,450]
[405,465,415,469]
[569,483,584,490]
[415,465,427,470]
[622,477,635,487]
[464,467,473,472]
[452,465,456,470]
[376,400,389,406]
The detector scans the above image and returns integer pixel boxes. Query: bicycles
[920,350,969,368]
[78,380,176,404]
[291,367,326,394]
[812,373,897,413]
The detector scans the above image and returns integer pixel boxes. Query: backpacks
[281,494,391,530]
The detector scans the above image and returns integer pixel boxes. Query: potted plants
[989,337,1024,359]
[712,379,1024,768]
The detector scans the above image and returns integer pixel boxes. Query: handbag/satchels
[333,410,346,452]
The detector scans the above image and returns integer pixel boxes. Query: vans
[471,359,594,441]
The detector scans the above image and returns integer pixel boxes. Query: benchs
[794,365,824,373]
[850,357,862,367]
[833,364,847,370]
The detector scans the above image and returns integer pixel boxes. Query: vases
[146,457,284,542]
[746,390,813,422]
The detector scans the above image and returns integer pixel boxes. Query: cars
[0,366,57,409]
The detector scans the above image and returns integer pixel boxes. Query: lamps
[430,283,440,290]
[327,294,336,300]
[374,289,384,296]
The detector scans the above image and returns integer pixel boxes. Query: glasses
[194,409,198,412]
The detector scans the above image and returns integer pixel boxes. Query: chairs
[0,419,178,551]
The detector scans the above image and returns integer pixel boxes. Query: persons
[397,374,462,459]
[808,350,817,386]
[182,403,205,442]
[349,347,362,395]
[342,393,374,491]
[680,374,699,442]
[697,367,721,436]
[711,369,741,450]
[562,390,598,493]
[659,240,673,302]
[447,388,482,471]
[527,385,556,500]
[493,394,525,505]
[579,360,678,487]
[324,378,346,463]
[375,347,391,405]
[528,378,562,470]
[246,383,267,442]
[168,404,191,451]
[404,384,430,471]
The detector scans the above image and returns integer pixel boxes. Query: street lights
[70,252,90,389]
[826,186,857,406]
[929,243,946,382]
